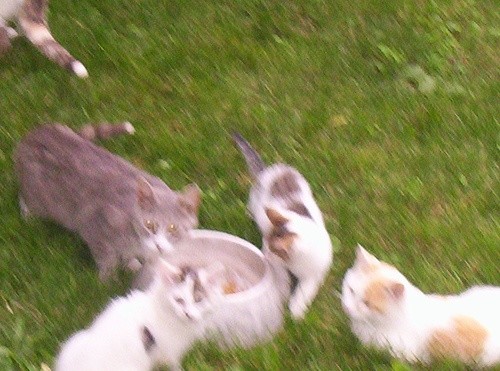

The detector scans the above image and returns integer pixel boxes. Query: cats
[228,125,334,323]
[339,243,500,371]
[0,0,90,79]
[13,120,206,289]
[54,256,226,371]
